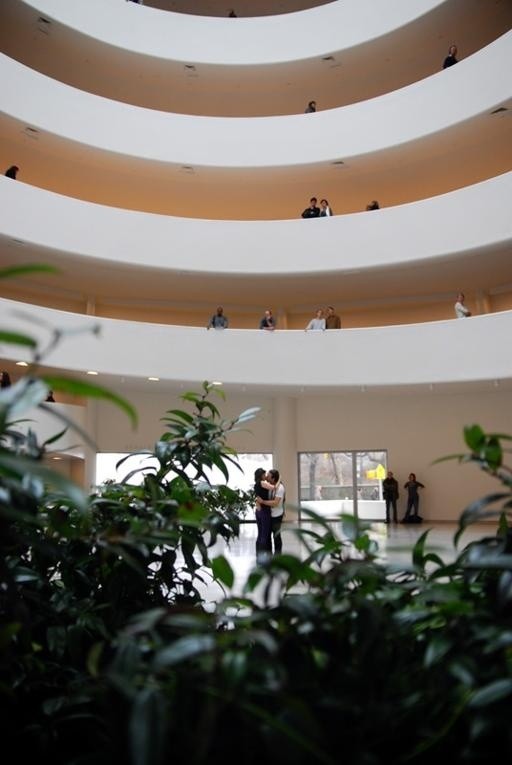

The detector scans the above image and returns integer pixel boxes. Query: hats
[255,468,266,481]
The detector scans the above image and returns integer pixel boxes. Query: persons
[228,9,237,17]
[206,306,228,331]
[257,309,276,331]
[319,199,333,216]
[4,165,19,180]
[304,100,316,113]
[305,308,327,330]
[254,468,287,556]
[301,197,320,218]
[404,472,425,521]
[454,291,473,319]
[367,200,379,210]
[325,305,342,329]
[443,45,457,69]
[382,471,400,523]
[251,467,285,557]
